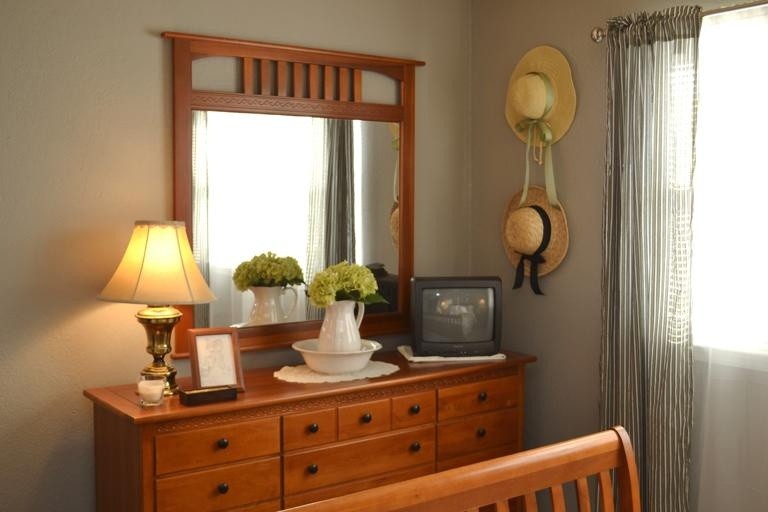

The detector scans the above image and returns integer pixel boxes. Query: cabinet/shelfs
[82,336,537,510]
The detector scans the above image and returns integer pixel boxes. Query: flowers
[232,250,306,293]
[304,260,390,312]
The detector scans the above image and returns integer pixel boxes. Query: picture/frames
[186,326,246,395]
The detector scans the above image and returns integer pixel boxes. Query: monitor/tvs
[410,276,502,356]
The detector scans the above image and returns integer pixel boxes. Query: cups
[138,375,166,407]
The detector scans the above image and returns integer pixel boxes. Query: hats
[500,185,569,277]
[506,45,577,150]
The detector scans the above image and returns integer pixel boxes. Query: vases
[248,287,298,323]
[317,300,365,352]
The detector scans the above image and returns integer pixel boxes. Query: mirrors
[162,29,426,360]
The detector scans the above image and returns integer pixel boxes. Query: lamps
[95,219,219,396]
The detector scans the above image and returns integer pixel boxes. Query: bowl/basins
[292,338,384,375]
